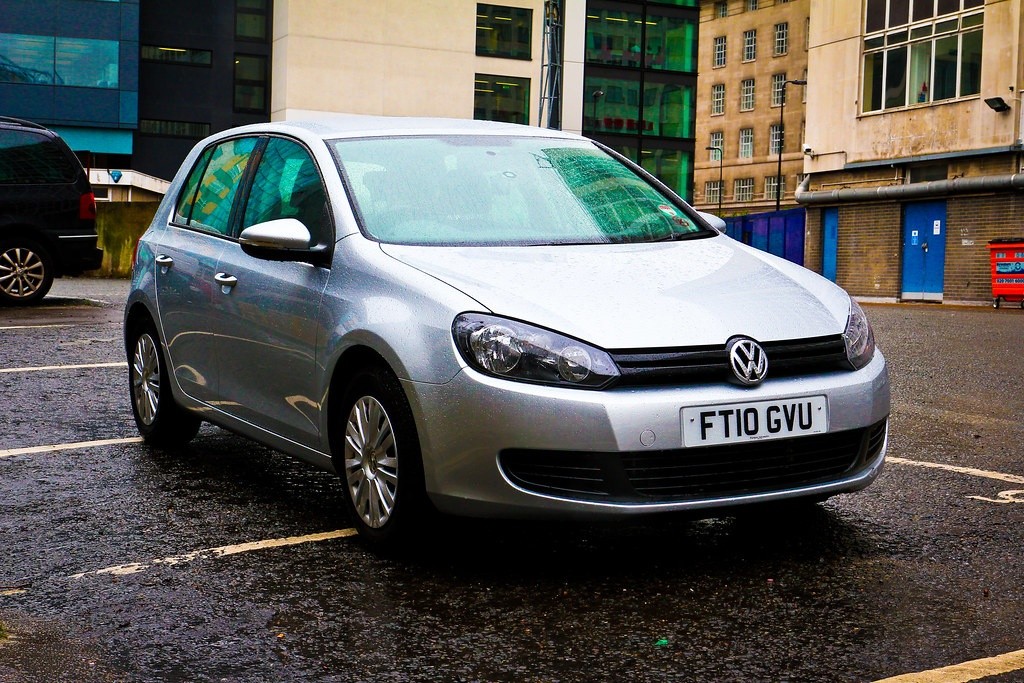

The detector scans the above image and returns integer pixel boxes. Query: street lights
[706,146,723,217]
[776,80,807,211]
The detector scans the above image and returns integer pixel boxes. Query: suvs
[0,113,97,309]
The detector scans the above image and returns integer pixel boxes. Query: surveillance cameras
[802,144,812,155]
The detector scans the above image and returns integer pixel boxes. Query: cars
[122,115,899,553]
